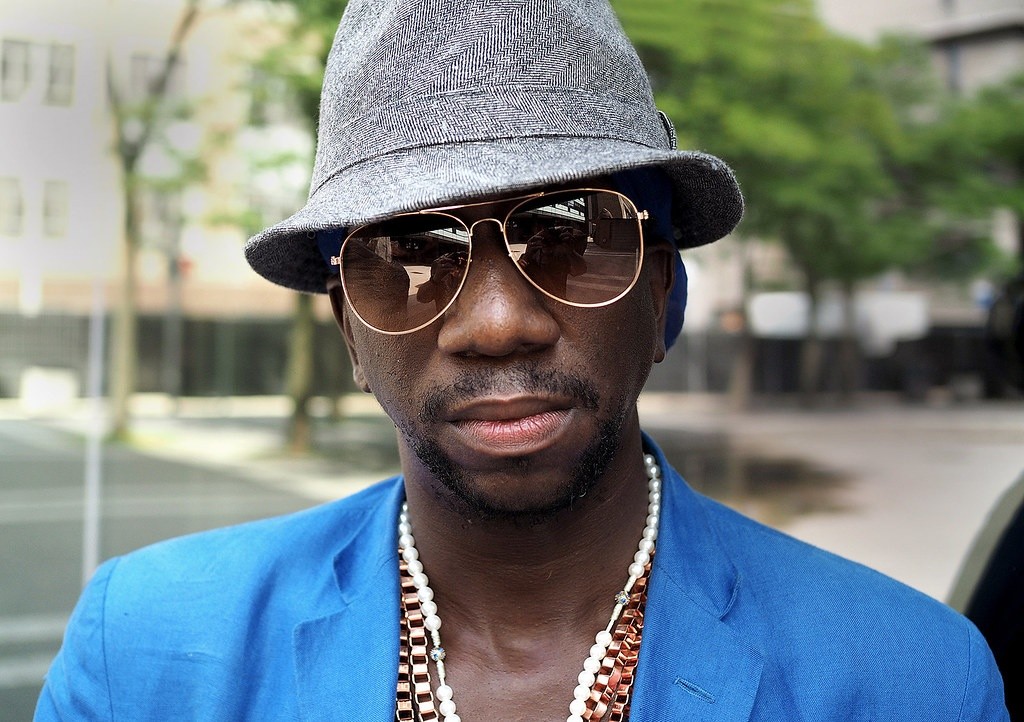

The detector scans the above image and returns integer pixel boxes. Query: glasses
[331,189,651,336]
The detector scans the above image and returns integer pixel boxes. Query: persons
[34,0,1010,722]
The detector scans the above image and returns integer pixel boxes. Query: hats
[245,0,745,292]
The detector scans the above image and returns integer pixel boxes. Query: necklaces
[396,453,661,722]
